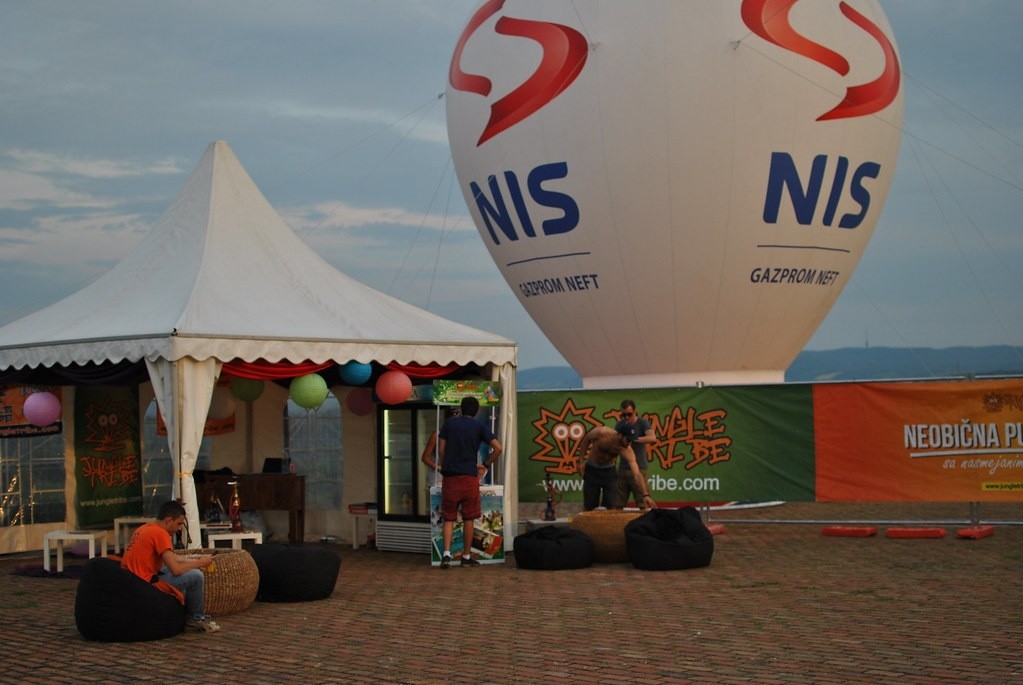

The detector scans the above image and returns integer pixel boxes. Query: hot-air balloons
[445,0,903,384]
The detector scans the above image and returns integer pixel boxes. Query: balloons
[23,392,61,427]
[376,370,412,404]
[232,377,265,402]
[289,373,327,408]
[346,387,373,417]
[339,360,372,385]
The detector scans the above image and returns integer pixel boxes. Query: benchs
[113,515,233,556]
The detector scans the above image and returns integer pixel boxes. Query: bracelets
[483,462,489,469]
[642,493,649,497]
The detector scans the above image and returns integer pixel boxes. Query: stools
[208,529,263,549]
[350,504,378,549]
[44,530,107,572]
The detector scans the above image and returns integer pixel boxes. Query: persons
[421,406,461,474]
[576,421,659,511]
[613,399,656,509]
[119,499,221,634]
[438,395,502,568]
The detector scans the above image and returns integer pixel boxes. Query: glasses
[622,409,634,418]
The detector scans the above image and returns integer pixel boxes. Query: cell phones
[213,550,218,556]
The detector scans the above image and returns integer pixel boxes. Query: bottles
[229,484,240,520]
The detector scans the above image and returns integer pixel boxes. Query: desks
[191,472,307,546]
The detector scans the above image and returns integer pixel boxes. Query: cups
[231,520,243,532]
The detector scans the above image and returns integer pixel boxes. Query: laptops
[261,457,291,476]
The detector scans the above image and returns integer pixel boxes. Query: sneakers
[460,556,479,567]
[186,615,220,634]
[440,555,450,568]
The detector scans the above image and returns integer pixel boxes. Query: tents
[0,138,518,553]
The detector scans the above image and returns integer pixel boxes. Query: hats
[619,423,638,441]
